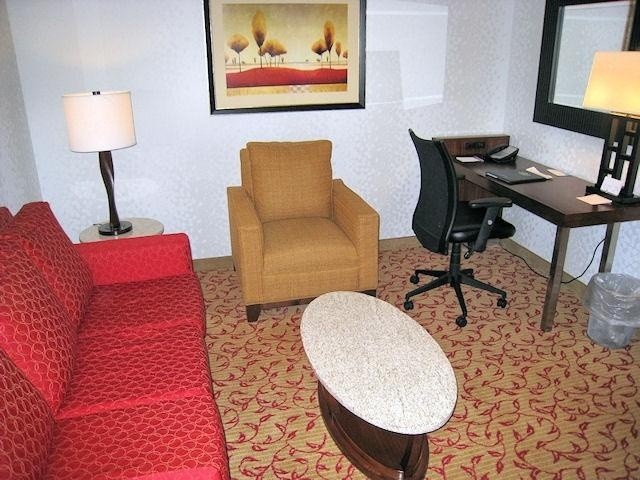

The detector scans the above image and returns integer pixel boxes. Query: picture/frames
[202,0,367,114]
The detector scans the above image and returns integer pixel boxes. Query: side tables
[76,215,162,242]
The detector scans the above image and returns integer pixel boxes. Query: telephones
[485,144,519,163]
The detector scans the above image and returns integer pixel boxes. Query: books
[485,167,546,185]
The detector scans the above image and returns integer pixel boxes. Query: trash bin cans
[587,272,640,349]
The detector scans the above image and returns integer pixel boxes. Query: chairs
[403,129,517,327]
[224,139,381,324]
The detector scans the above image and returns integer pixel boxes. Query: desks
[459,146,640,337]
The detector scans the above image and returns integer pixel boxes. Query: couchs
[0,193,233,478]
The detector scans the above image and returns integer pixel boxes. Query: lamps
[579,45,640,207]
[60,87,137,240]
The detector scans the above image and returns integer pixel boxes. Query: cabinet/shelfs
[432,134,511,219]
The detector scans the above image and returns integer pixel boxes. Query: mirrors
[532,0,640,146]
